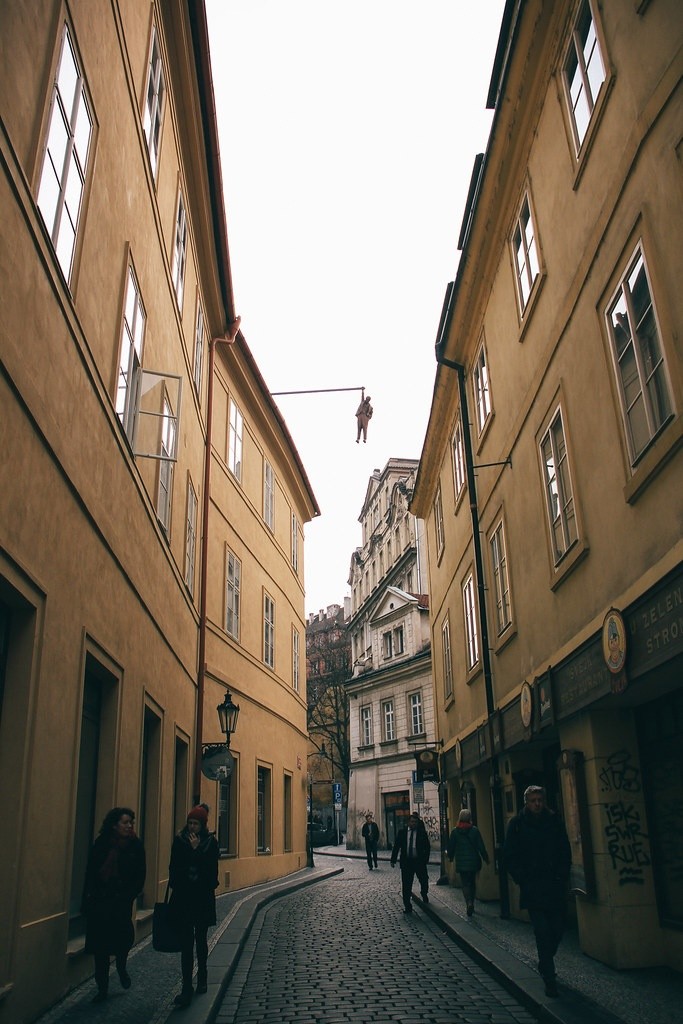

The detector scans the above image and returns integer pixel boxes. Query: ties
[409,829,415,857]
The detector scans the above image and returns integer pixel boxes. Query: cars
[307,822,345,846]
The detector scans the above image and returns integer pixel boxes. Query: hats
[187,803,209,829]
[459,808,472,822]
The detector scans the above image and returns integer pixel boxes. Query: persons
[503,785,572,998]
[446,809,490,915]
[81,808,146,1001]
[356,388,373,443]
[391,811,430,913]
[362,815,379,869]
[168,803,218,1006]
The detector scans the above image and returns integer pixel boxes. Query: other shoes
[91,991,109,1008]
[174,989,194,1006]
[466,899,474,917]
[196,969,207,994]
[419,892,429,903]
[541,975,559,999]
[117,965,131,989]
[403,904,412,913]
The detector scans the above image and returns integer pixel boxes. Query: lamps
[203,688,242,756]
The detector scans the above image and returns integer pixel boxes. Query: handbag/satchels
[477,854,483,870]
[152,880,183,952]
